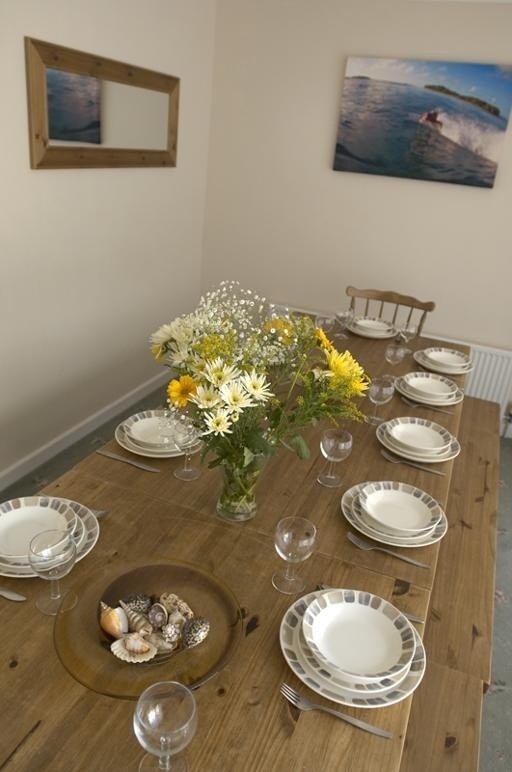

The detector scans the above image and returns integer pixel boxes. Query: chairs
[345,286,434,336]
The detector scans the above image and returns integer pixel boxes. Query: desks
[76,313,471,591]
[0,467,471,772]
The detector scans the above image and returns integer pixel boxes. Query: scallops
[111,634,160,664]
[183,617,210,650]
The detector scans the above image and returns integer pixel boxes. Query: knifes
[1,586,28,602]
[95,448,161,473]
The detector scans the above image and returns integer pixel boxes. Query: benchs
[400,660,483,772]
[420,396,499,692]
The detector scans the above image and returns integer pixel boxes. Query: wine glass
[334,307,354,340]
[400,322,418,354]
[385,344,405,375]
[28,529,79,615]
[366,378,395,426]
[173,420,202,482]
[316,429,353,488]
[132,680,200,772]
[270,516,317,596]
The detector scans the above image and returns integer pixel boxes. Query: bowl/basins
[386,416,451,454]
[403,371,459,399]
[123,409,192,448]
[302,590,417,684]
[0,496,79,564]
[359,480,443,537]
[424,347,472,368]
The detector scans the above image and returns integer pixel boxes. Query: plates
[298,620,414,694]
[340,481,448,548]
[352,494,436,540]
[413,350,475,376]
[114,418,203,459]
[280,587,427,709]
[376,417,461,463]
[1,497,100,579]
[395,376,464,406]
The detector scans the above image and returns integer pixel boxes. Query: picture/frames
[23,36,178,168]
[332,55,511,189]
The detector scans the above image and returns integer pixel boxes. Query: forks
[279,681,394,739]
[379,449,447,476]
[345,530,432,569]
[401,396,455,415]
[316,583,425,624]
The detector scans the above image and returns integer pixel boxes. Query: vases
[216,454,264,522]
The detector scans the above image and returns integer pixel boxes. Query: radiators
[269,305,512,437]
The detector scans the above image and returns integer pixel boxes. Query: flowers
[148,279,370,503]
